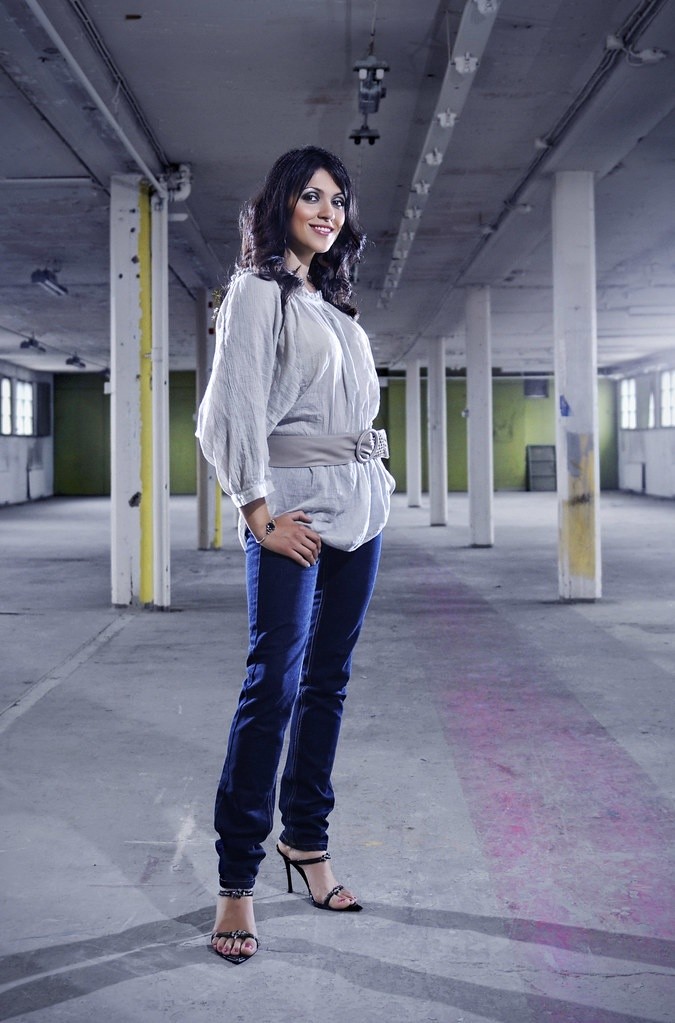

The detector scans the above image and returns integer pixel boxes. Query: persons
[183,146,399,966]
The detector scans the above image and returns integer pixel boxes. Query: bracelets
[255,516,277,545]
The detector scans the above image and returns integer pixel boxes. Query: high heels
[208,890,263,965]
[275,844,363,911]
